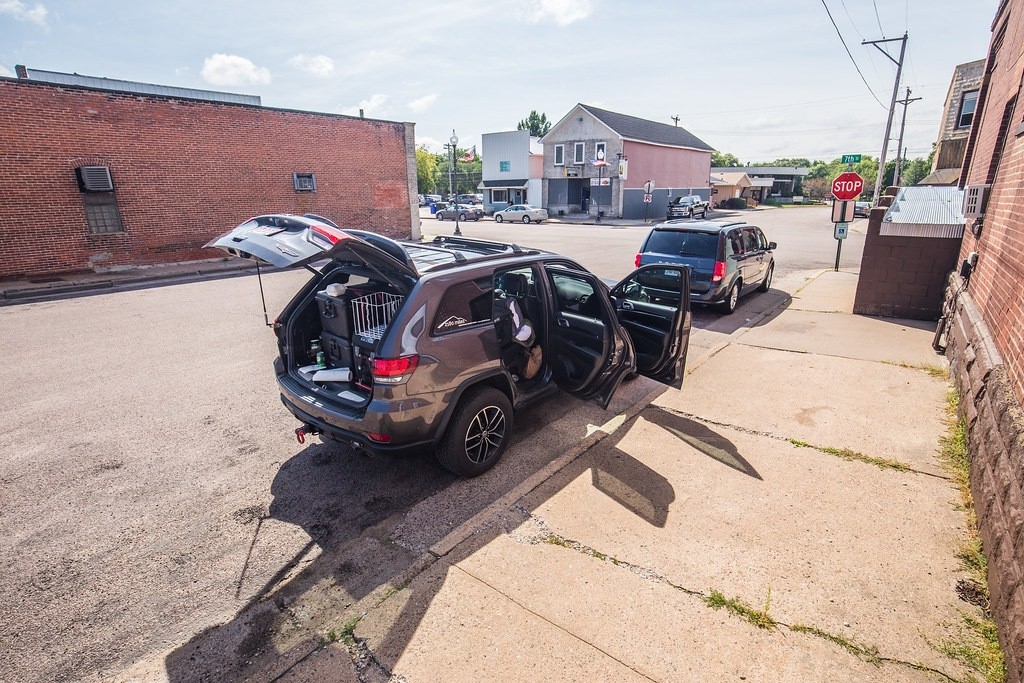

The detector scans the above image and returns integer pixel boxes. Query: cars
[418,194,425,208]
[202,211,694,480]
[436,204,485,222]
[493,204,548,224]
[853,202,871,219]
[449,194,477,205]
[475,194,482,204]
[424,197,438,205]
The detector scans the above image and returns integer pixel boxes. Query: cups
[310,339,319,350]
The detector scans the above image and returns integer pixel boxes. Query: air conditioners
[293,173,317,190]
[79,165,113,190]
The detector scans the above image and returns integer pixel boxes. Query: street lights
[450,129,463,236]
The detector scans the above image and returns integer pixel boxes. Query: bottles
[316,351,326,368]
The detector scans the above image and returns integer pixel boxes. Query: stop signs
[831,172,864,202]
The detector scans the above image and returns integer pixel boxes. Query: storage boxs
[351,344,374,392]
[320,332,352,371]
[351,292,405,340]
[317,290,369,338]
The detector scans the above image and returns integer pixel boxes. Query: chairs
[499,272,539,337]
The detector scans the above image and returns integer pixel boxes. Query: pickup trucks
[666,194,710,220]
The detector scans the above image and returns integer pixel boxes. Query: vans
[635,217,778,315]
